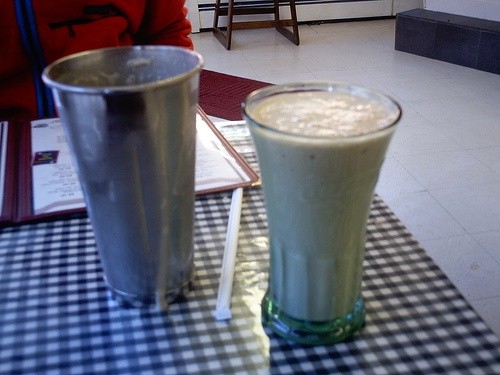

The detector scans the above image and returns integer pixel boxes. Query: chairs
[212,0,300,51]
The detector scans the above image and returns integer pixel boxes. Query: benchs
[394,8,500,74]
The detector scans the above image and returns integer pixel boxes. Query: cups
[41,45,205,307]
[241,81,403,346]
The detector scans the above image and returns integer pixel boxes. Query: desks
[1,114,500,375]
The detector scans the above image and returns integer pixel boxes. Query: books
[0,102,262,225]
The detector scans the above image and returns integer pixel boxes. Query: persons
[0,0,195,120]
